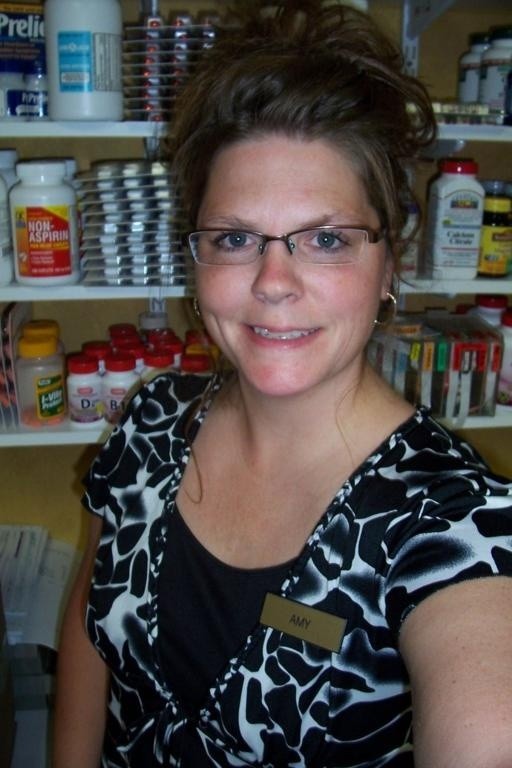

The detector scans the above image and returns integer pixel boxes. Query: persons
[50,1,512,768]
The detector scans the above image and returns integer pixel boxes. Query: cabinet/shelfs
[0,124,510,449]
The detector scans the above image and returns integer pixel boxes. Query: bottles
[0,2,124,285]
[14,317,227,432]
[422,27,512,409]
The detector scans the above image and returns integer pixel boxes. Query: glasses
[187,224,386,264]
